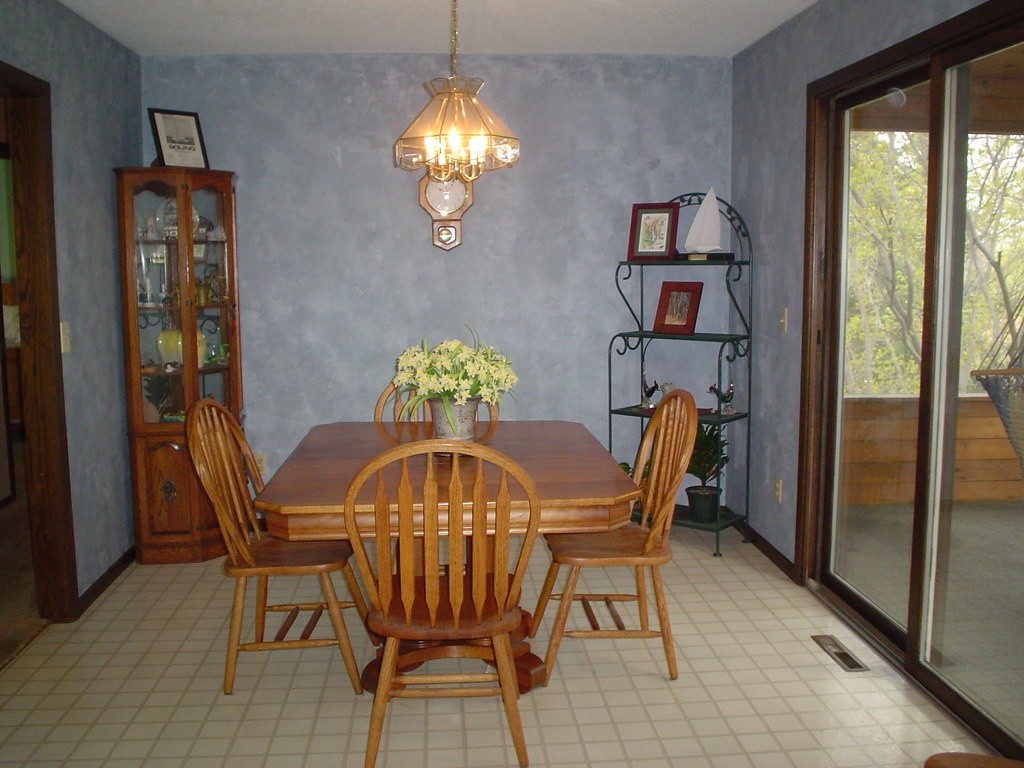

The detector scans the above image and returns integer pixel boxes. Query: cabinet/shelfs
[608,193,754,556]
[111,166,248,566]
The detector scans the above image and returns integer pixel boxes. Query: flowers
[385,321,519,436]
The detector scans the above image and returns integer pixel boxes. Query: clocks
[419,172,474,251]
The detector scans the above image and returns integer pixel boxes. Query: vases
[157,321,181,367]
[425,397,482,458]
[178,329,207,369]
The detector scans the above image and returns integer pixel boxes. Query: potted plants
[686,422,731,524]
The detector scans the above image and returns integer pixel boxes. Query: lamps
[395,0,521,183]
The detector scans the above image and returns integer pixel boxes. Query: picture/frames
[627,202,680,261]
[147,107,211,169]
[653,281,704,335]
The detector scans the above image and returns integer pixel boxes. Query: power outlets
[774,479,782,505]
[254,453,267,476]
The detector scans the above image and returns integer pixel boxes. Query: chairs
[528,389,697,680]
[375,382,501,422]
[185,397,381,697]
[343,439,542,768]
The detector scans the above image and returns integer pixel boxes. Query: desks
[253,422,646,695]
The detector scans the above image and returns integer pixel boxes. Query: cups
[197,282,211,304]
